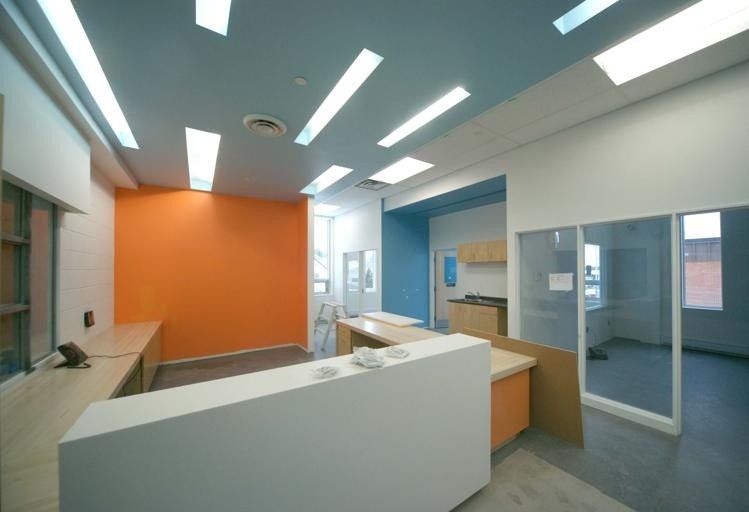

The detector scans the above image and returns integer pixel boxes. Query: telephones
[56,340,89,366]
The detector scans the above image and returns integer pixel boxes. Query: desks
[1,322,162,512]
[335,316,539,453]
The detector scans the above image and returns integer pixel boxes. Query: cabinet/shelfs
[447,302,507,335]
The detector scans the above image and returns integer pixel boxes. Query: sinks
[471,300,482,304]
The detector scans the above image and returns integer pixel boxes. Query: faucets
[467,290,480,298]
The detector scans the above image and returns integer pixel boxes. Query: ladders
[314,300,351,351]
